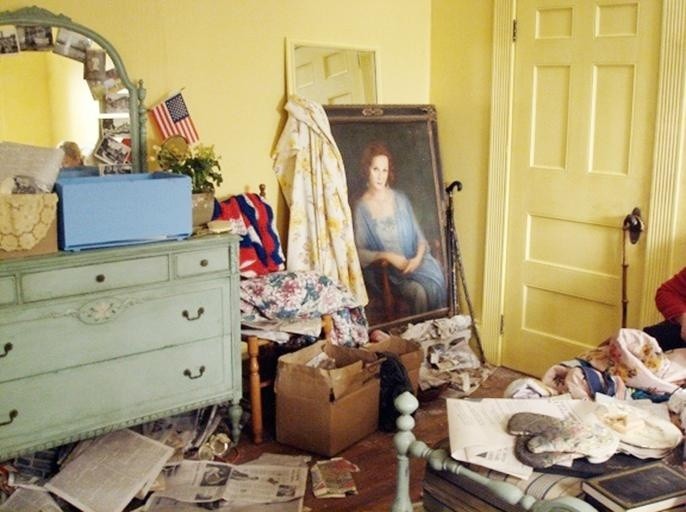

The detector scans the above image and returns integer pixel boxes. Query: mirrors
[0,6,145,171]
[285,40,381,110]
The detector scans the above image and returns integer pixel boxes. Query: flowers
[152,142,222,192]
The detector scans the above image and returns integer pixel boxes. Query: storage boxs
[0,172,192,260]
[275,336,423,457]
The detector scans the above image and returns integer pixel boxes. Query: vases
[191,192,215,234]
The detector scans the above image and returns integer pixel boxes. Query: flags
[148,92,199,145]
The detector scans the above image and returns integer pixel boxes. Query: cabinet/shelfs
[0,229,243,464]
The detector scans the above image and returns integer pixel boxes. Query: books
[581,460,686,512]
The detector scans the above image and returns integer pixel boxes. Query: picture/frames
[320,105,460,332]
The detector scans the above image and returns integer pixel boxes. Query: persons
[352,140,448,315]
[638,267,686,351]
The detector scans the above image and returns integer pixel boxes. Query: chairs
[208,184,330,446]
